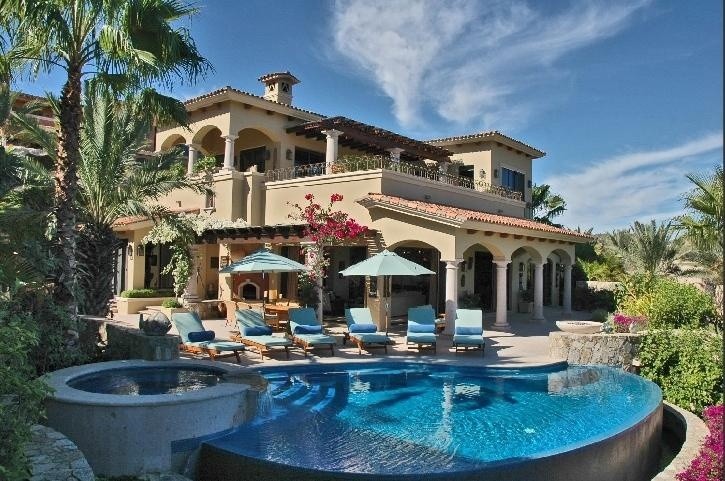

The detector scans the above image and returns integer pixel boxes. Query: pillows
[349,324,377,333]
[245,326,272,336]
[409,325,434,333]
[456,327,482,335]
[294,325,322,334]
[188,331,212,342]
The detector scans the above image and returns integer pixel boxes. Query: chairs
[343,307,395,355]
[288,308,336,358]
[406,308,437,354]
[454,309,484,353]
[416,304,446,333]
[223,298,300,331]
[234,309,292,361]
[172,311,245,363]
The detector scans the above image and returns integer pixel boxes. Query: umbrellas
[337,248,437,337]
[219,248,308,318]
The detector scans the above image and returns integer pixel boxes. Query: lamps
[127,245,132,255]
[137,244,144,255]
